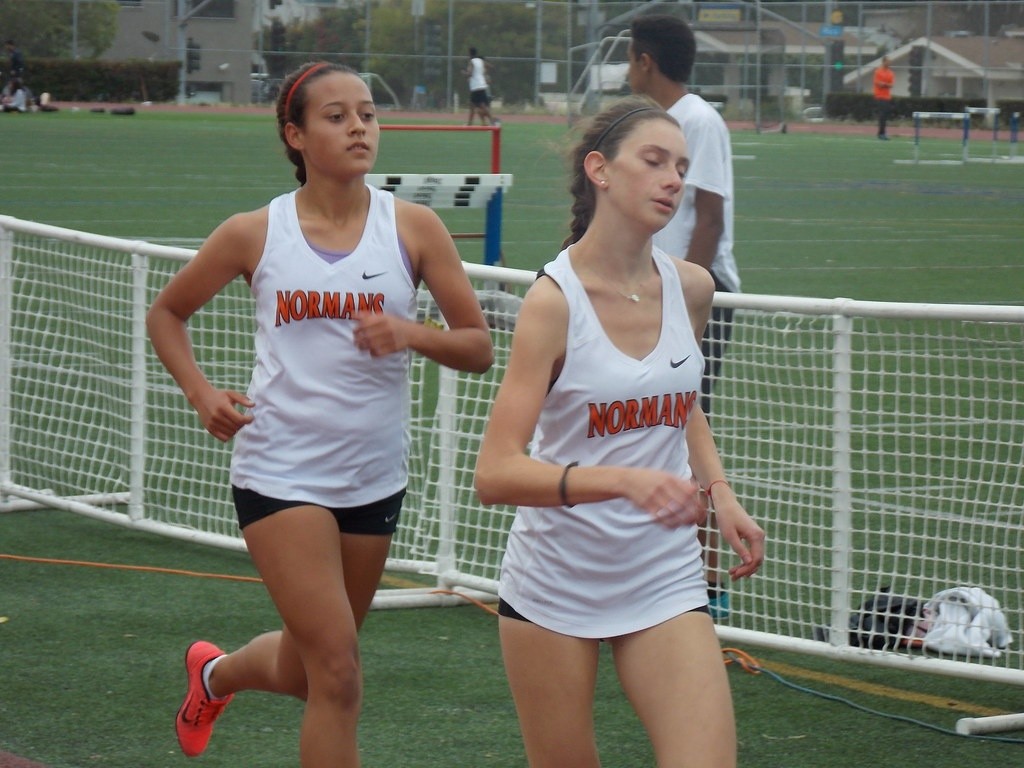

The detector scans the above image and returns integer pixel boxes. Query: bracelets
[559,460,583,510]
[706,480,729,496]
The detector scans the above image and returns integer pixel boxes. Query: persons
[147,60,495,768]
[0,39,36,114]
[473,89,772,768]
[459,48,498,128]
[619,14,742,596]
[873,56,895,140]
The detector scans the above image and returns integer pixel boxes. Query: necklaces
[572,230,655,302]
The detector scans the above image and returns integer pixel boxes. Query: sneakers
[175,640,236,759]
